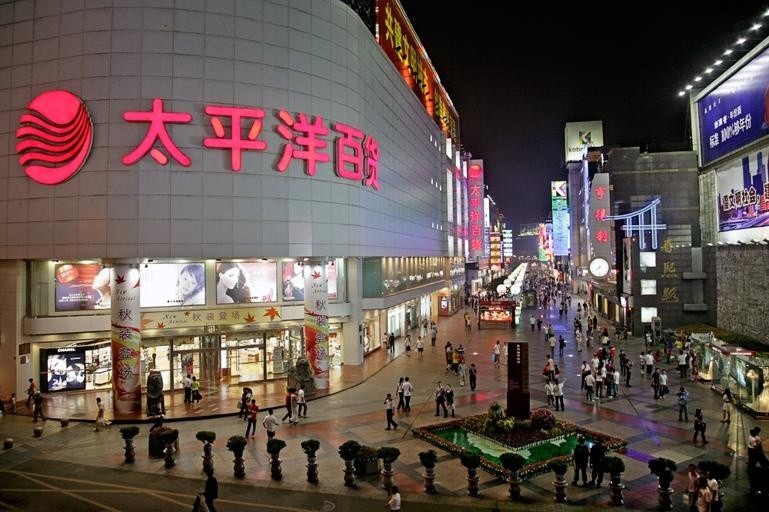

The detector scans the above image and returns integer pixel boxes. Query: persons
[720,387,734,423]
[675,386,689,422]
[640,325,699,400]
[542,355,567,412]
[685,463,724,511]
[48,355,66,390]
[176,264,206,306]
[745,427,767,470]
[263,408,280,441]
[571,437,607,488]
[464,291,509,314]
[692,407,709,444]
[297,384,309,417]
[238,387,252,420]
[464,311,472,333]
[382,318,438,357]
[530,315,567,357]
[384,377,413,430]
[93,397,106,430]
[204,468,219,511]
[217,263,253,304]
[192,494,210,511]
[521,262,572,320]
[0,392,16,414]
[289,388,299,423]
[445,341,477,392]
[243,398,259,439]
[434,381,456,419]
[574,299,628,352]
[581,346,634,402]
[92,266,111,309]
[24,378,38,406]
[181,362,202,405]
[283,260,305,300]
[384,485,401,510]
[32,390,48,422]
[282,388,292,422]
[493,340,508,369]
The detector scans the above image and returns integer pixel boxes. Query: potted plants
[496,452,527,501]
[696,459,732,484]
[147,425,181,469]
[225,434,248,478]
[644,456,676,508]
[119,425,140,465]
[195,429,217,475]
[265,438,287,481]
[337,438,401,490]
[546,458,570,503]
[598,454,626,506]
[459,450,484,499]
[416,448,441,494]
[300,438,321,484]
